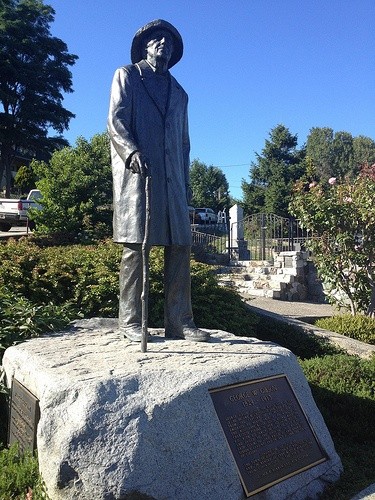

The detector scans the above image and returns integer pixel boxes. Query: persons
[105,18,211,342]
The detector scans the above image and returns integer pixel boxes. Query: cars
[195,208,217,224]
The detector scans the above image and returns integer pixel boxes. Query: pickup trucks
[0,189,46,231]
